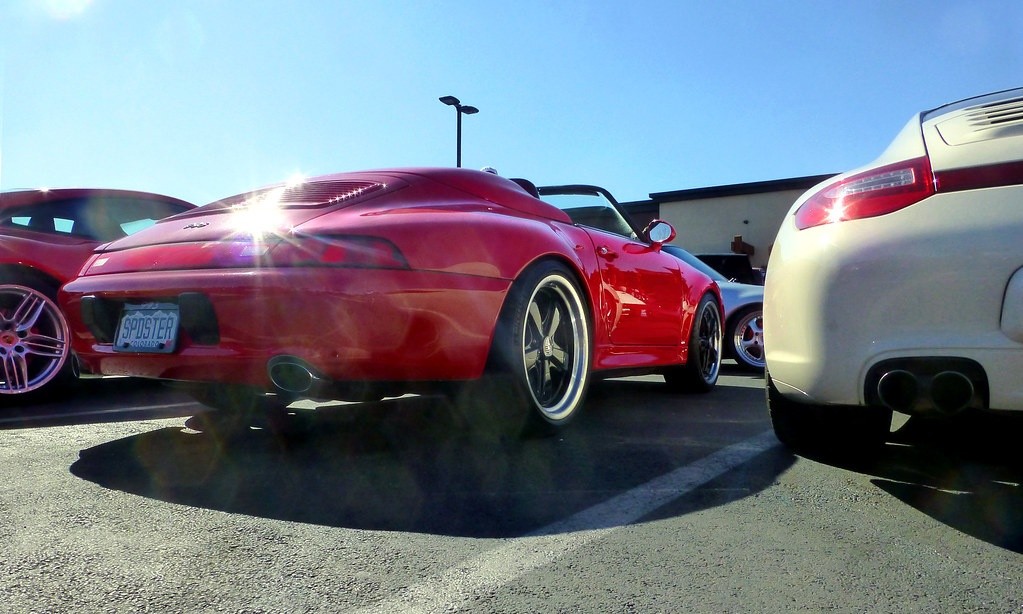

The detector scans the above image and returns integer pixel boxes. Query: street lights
[441,96,480,170]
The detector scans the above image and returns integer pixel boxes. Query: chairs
[70,208,94,238]
[28,214,55,232]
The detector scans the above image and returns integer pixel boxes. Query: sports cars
[0,167,768,438]
[760,87,1023,456]
